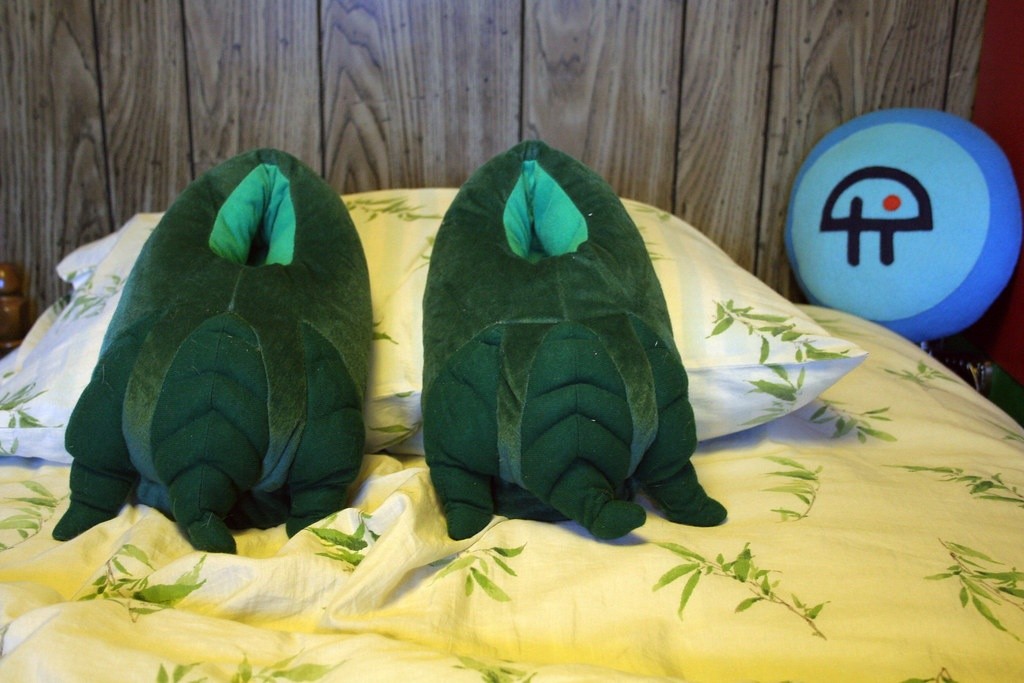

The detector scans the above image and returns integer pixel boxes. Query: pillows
[0,184,867,467]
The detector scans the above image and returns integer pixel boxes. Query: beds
[0,290,1024,683]
